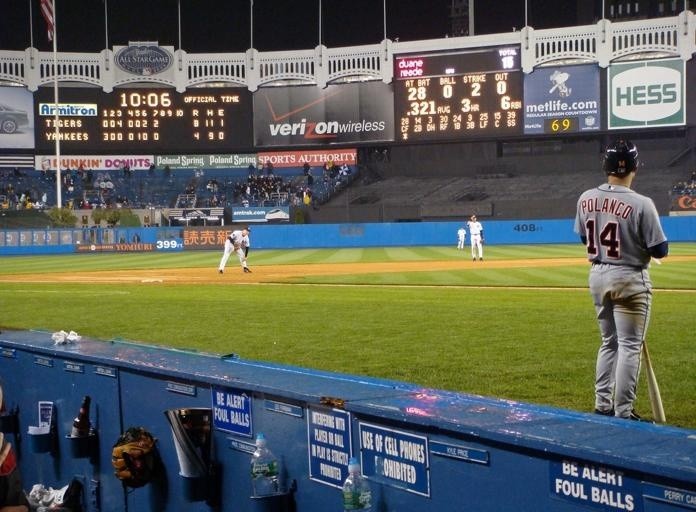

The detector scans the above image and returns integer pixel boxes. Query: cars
[0,102,28,134]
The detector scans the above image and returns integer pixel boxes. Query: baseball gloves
[234,241,240,250]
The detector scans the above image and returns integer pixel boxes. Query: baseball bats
[643,339,667,422]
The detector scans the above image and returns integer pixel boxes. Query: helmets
[603,139,640,177]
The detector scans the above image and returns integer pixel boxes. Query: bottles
[72,396,90,436]
[341,457,373,512]
[251,433,280,499]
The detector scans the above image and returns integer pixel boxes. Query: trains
[264,208,289,220]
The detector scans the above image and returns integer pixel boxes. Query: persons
[1,165,57,209]
[234,160,313,208]
[148,162,169,177]
[184,179,231,209]
[574,139,668,421]
[466,214,484,262]
[323,160,348,185]
[218,226,251,273]
[457,227,466,253]
[61,165,131,208]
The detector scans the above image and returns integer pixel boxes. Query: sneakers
[594,408,655,424]
[25,478,79,511]
